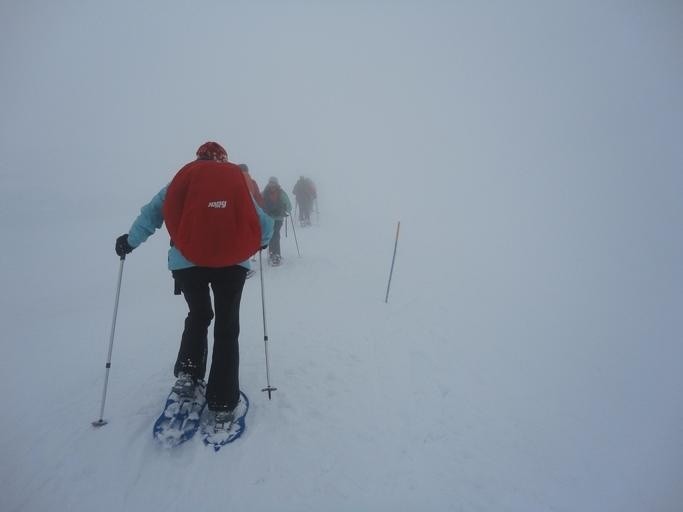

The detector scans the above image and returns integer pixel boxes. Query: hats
[196,142,227,161]
[238,164,249,173]
[268,177,277,184]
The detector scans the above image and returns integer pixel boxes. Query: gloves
[116,234,134,255]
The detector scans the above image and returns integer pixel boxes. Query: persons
[260,176,291,264]
[114,142,273,422]
[239,163,266,210]
[291,174,320,226]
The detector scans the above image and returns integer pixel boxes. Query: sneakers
[172,373,199,398]
[270,253,273,259]
[273,255,281,263]
[216,410,234,423]
